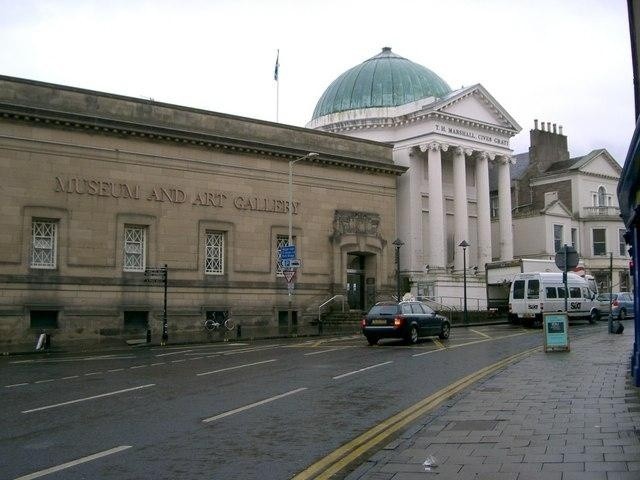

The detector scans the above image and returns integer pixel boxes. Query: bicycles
[205,309,236,332]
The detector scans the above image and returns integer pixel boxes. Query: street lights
[287,151,319,310]
[458,240,471,324]
[393,238,404,302]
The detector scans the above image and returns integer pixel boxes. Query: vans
[508,272,601,328]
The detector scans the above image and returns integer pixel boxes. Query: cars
[597,292,635,320]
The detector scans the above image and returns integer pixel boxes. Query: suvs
[362,300,451,345]
